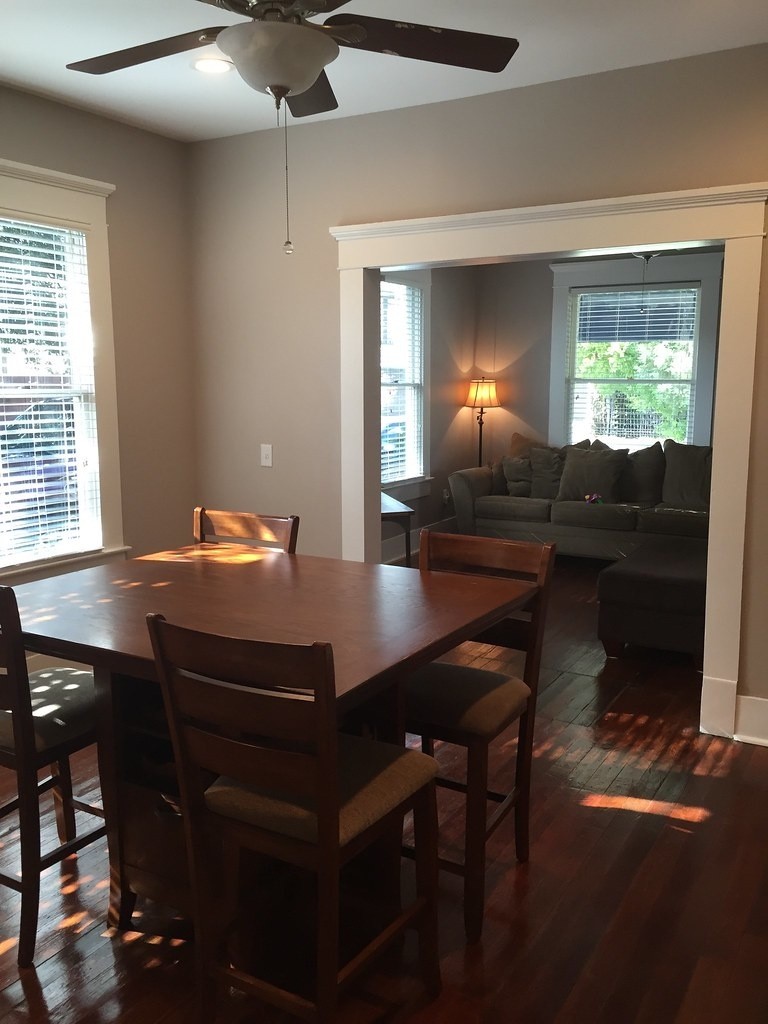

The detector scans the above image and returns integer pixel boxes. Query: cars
[0,397,79,537]
[381,422,405,469]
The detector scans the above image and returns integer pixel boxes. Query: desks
[380,491,416,568]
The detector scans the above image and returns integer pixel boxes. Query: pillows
[501,434,713,511]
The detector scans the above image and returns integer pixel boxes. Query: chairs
[0,586,106,967]
[191,506,301,553]
[145,612,446,1024]
[349,528,557,942]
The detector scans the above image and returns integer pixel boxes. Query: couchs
[447,433,713,561]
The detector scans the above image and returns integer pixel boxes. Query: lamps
[214,12,340,127]
[465,376,502,467]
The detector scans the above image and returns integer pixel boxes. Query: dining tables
[10,542,539,971]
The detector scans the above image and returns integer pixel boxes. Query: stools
[595,537,709,675]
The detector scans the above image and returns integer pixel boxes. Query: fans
[66,0,520,254]
